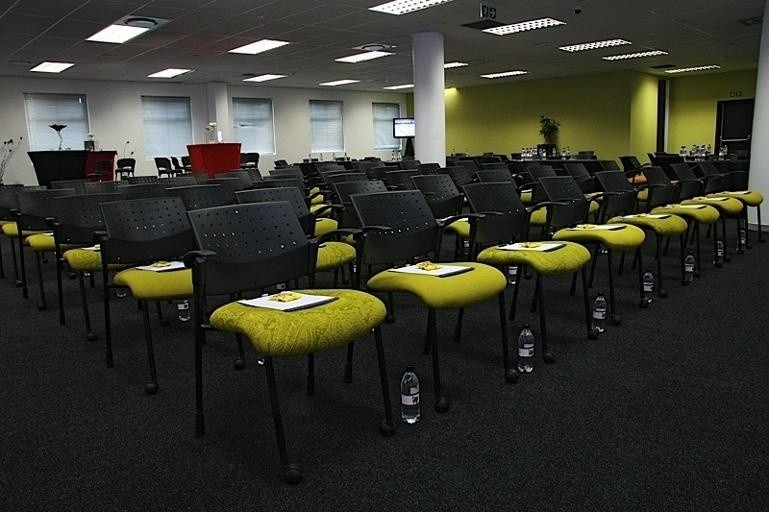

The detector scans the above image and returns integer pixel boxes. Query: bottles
[717,239,724,263]
[680,144,712,162]
[592,291,607,332]
[685,251,695,281]
[508,266,518,284]
[739,227,746,251]
[517,323,535,373]
[391,151,402,161]
[562,146,570,161]
[308,153,347,163]
[401,365,421,424]
[177,299,191,322]
[719,145,728,161]
[116,287,127,297]
[463,240,470,255]
[521,147,546,162]
[553,148,556,157]
[643,268,654,303]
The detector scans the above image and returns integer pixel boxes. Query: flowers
[205,122,217,132]
[87,132,95,141]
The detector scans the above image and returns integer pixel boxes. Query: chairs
[0,146,764,341]
[344,188,523,415]
[181,199,400,486]
[454,182,602,366]
[99,197,247,397]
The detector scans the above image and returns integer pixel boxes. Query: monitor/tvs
[393,118,415,138]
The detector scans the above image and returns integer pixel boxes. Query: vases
[208,131,216,144]
